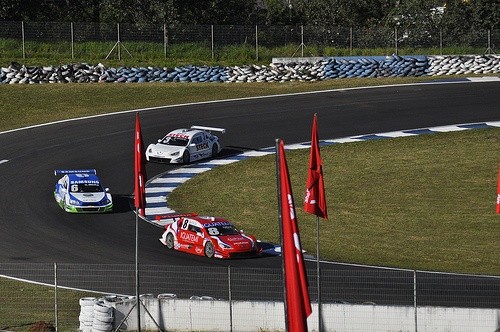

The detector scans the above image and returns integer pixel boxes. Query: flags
[277,140,313,331]
[133,110,147,218]
[495,162,500,215]
[303,112,329,220]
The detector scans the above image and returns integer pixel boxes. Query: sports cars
[53,169,114,213]
[155,212,263,259]
[145,125,226,165]
